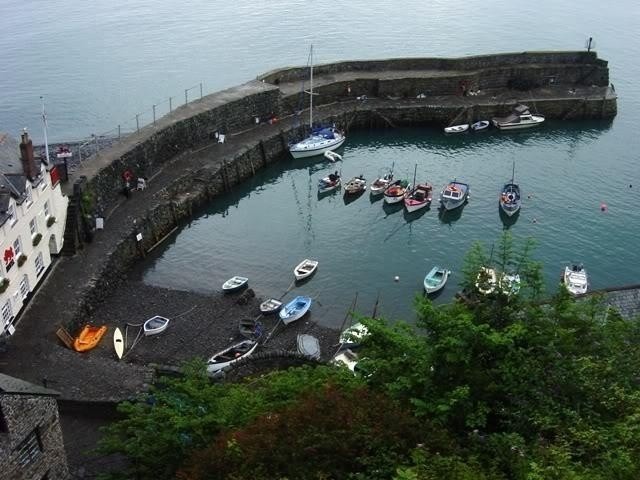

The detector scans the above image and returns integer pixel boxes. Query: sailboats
[288,44,348,159]
[499,159,522,215]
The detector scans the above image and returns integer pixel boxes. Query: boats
[423,266,451,295]
[73,325,107,353]
[113,327,125,359]
[499,271,521,302]
[563,261,588,298]
[317,158,470,212]
[477,267,496,294]
[143,315,170,337]
[445,103,546,134]
[201,257,377,384]
